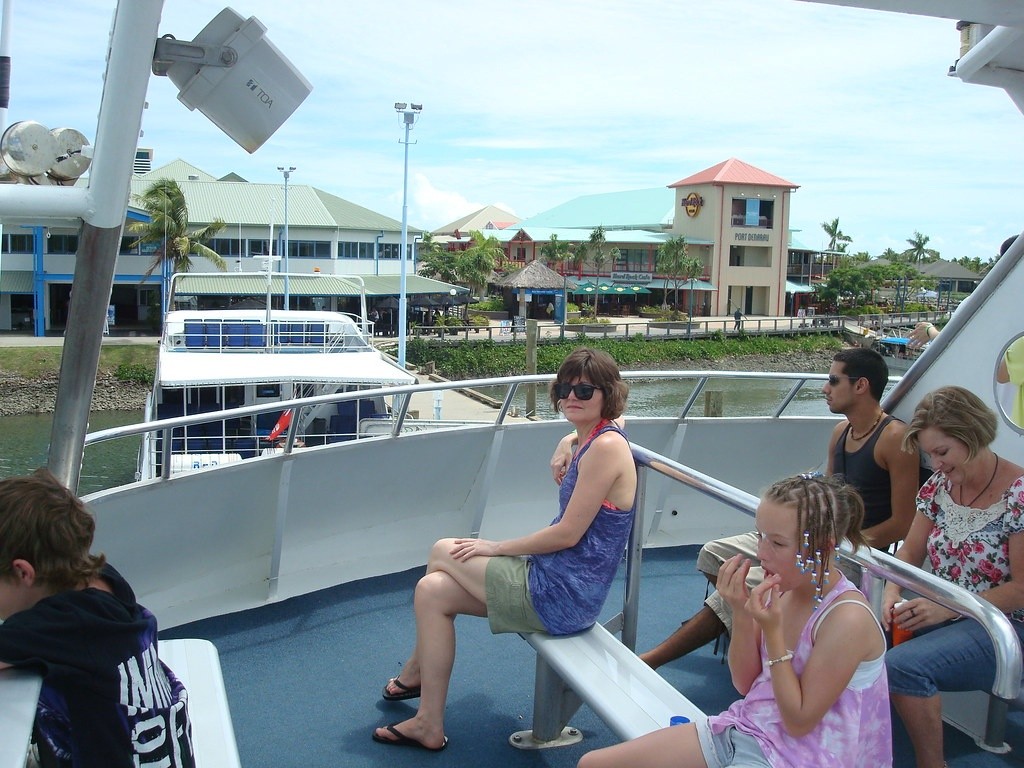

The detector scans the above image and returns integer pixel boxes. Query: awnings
[786,281,828,293]
[565,276,718,292]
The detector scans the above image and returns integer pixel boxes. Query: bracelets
[951,614,963,621]
[572,429,577,434]
[765,652,794,667]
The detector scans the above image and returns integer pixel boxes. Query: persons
[734,308,742,331]
[370,307,440,337]
[637,346,923,673]
[372,346,641,751]
[575,472,896,768]
[1,468,196,768]
[878,385,1024,768]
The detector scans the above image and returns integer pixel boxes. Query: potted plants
[473,315,491,333]
[145,303,162,334]
[411,322,423,339]
[435,315,464,337]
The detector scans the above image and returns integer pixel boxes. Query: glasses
[828,375,859,386]
[556,382,604,400]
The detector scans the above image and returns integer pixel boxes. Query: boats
[131,198,419,483]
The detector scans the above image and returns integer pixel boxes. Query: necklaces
[959,452,998,508]
[850,408,883,441]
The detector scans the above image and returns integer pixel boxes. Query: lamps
[152,7,314,154]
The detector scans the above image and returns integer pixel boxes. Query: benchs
[158,639,242,768]
[510,620,710,750]
[468,317,490,330]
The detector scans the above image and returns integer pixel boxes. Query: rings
[910,608,917,618]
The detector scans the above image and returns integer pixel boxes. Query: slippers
[382,674,421,701]
[372,720,448,751]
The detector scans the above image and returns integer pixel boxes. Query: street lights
[394,101,423,370]
[449,288,456,318]
[277,166,297,310]
[687,276,697,334]
[790,288,796,330]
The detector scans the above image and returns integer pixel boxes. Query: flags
[268,408,293,441]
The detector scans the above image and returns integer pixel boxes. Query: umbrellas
[376,293,479,338]
[573,281,652,307]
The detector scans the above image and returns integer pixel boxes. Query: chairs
[184,319,345,349]
[157,402,389,459]
